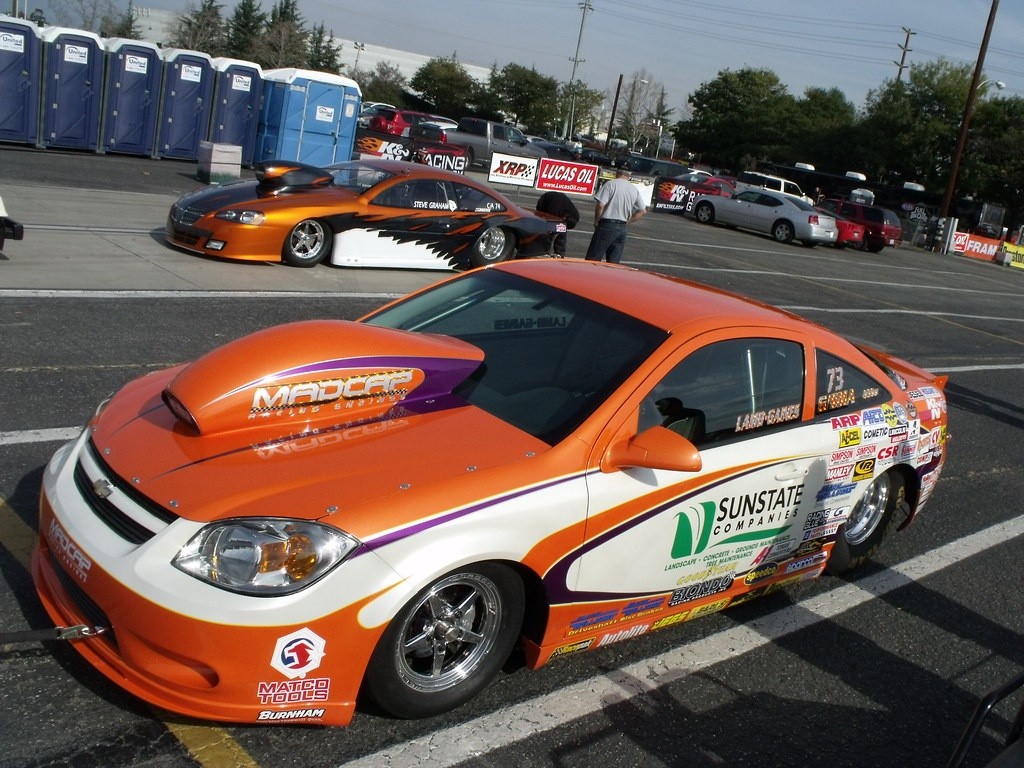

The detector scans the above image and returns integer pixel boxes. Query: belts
[601,219,626,224]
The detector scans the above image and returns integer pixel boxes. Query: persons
[537,192,579,258]
[585,162,646,264]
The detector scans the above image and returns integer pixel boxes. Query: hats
[617,161,632,172]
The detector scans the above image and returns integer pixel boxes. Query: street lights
[932,80,1006,253]
[351,41,367,79]
[603,74,649,153]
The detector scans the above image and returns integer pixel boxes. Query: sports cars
[32,255,954,726]
[166,157,569,273]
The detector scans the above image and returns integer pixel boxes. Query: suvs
[736,169,818,209]
[368,107,441,138]
[815,198,905,254]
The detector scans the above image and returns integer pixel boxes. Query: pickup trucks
[408,117,551,171]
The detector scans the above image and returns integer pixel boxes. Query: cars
[656,172,736,211]
[813,206,866,249]
[691,188,839,248]
[522,135,548,143]
[536,140,576,163]
[357,101,397,114]
[581,146,618,169]
[416,110,460,131]
[614,155,632,172]
[688,167,714,178]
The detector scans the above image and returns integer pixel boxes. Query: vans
[622,151,689,188]
